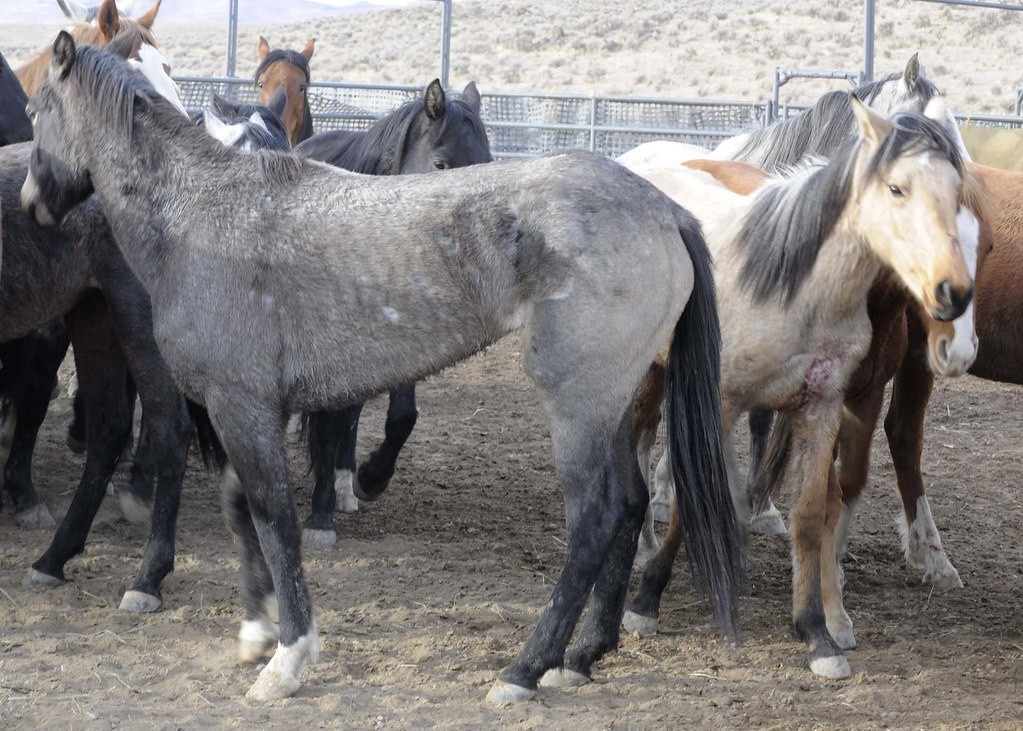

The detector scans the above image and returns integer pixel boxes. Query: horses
[15,30,748,710]
[613,94,976,681]
[0,1,1023,665]
